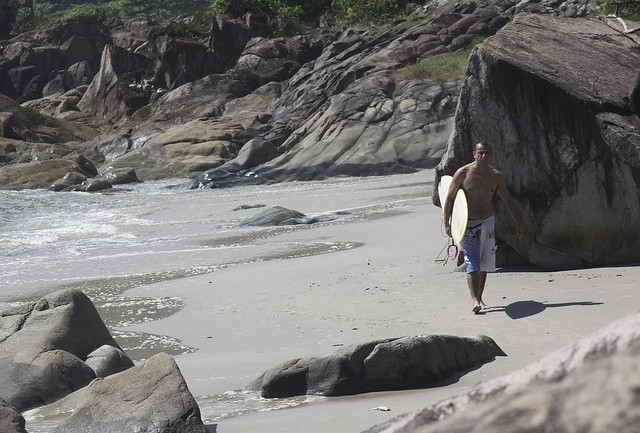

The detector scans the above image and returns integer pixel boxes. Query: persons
[444,142,526,316]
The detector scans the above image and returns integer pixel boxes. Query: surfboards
[437,175,468,247]
